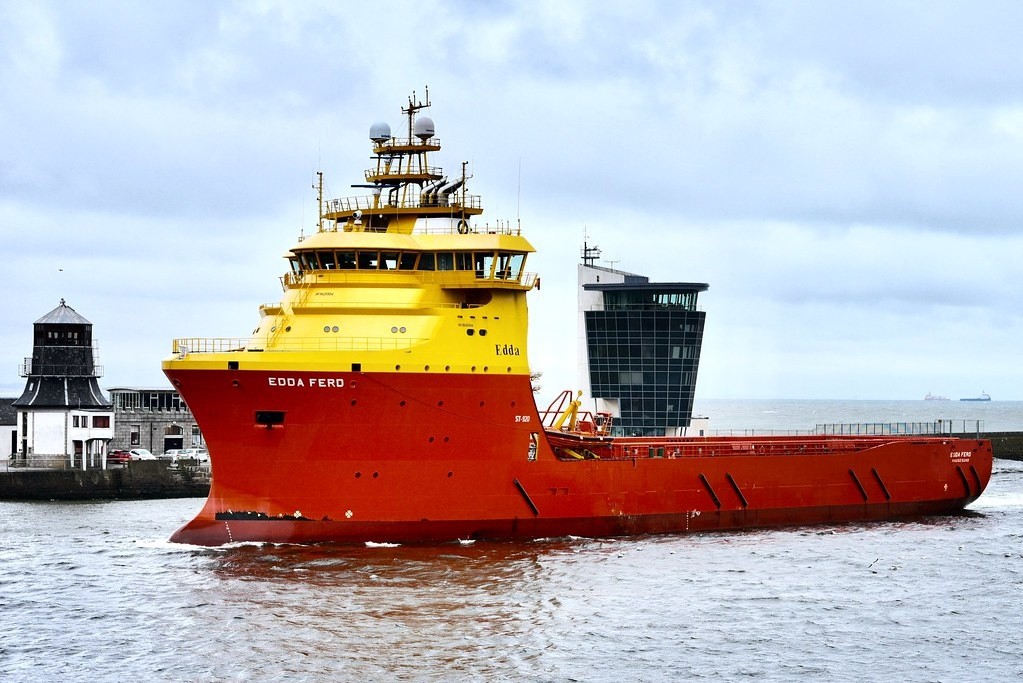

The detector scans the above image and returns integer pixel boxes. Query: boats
[161,85,995,556]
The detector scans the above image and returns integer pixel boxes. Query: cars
[158,448,191,460]
[128,449,157,461]
[107,448,132,464]
[186,449,208,462]
